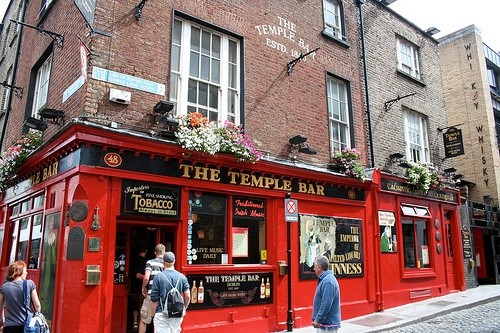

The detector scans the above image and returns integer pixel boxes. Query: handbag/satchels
[23,311,49,333]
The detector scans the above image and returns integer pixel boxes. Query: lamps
[39,108,64,119]
[158,112,179,126]
[289,135,307,145]
[23,117,47,131]
[153,101,174,115]
[444,168,456,173]
[391,153,403,160]
[452,175,464,181]
[381,0,397,5]
[426,27,440,36]
[298,144,317,154]
[398,160,412,168]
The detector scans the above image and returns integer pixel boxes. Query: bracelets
[150,314,155,318]
[142,286,146,288]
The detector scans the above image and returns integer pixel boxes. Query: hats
[163,252,175,263]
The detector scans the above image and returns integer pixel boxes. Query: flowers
[409,160,447,193]
[174,112,262,162]
[333,148,365,179]
[0,129,43,193]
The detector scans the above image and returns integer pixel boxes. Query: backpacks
[160,271,185,318]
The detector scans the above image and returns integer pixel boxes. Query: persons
[311,256,341,333]
[131,247,146,329]
[0,260,41,333]
[139,243,168,333]
[149,252,191,333]
